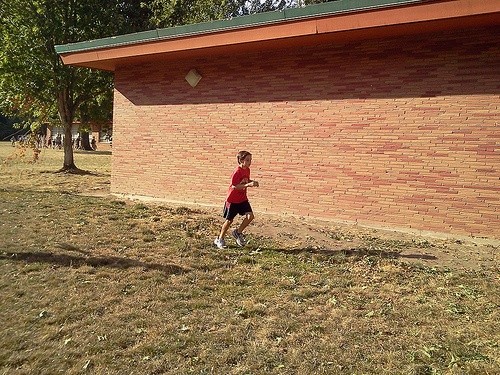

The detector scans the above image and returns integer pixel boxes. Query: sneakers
[231,229,245,247]
[214,238,225,249]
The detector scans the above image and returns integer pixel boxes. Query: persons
[10,132,112,152]
[214,150,260,249]
